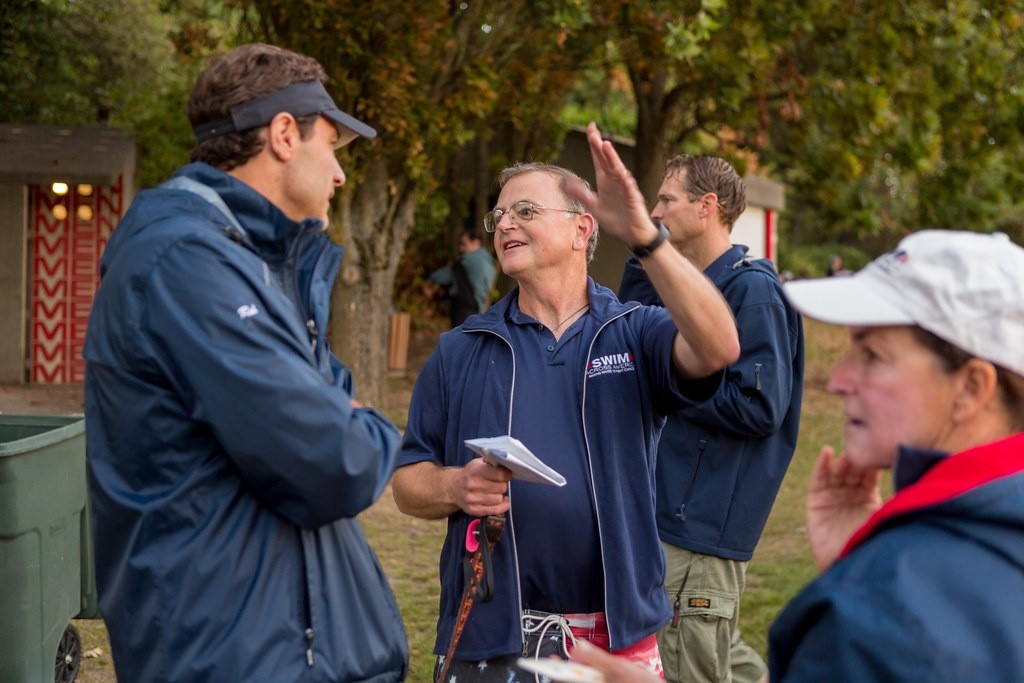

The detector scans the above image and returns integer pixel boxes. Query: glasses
[482,200,582,233]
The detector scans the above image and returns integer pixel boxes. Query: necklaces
[550,303,594,332]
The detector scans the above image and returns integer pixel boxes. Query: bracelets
[624,221,670,259]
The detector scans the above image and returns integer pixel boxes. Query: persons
[391,120,741,683]
[546,227,1024,683]
[429,224,495,330]
[84,41,411,683]
[617,151,805,683]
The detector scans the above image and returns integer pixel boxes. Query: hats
[194,79,377,150]
[782,229,1024,376]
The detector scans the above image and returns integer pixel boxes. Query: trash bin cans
[0,410,98,682]
